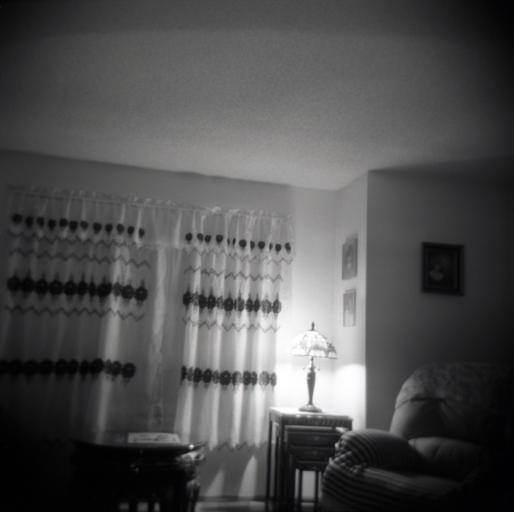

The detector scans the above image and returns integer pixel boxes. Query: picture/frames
[342,289,356,329]
[421,242,465,296]
[341,238,357,280]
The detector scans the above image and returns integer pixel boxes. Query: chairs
[321,361,513,512]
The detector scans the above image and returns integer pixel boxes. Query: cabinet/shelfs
[265,407,352,511]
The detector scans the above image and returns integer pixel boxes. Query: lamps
[286,322,337,412]
